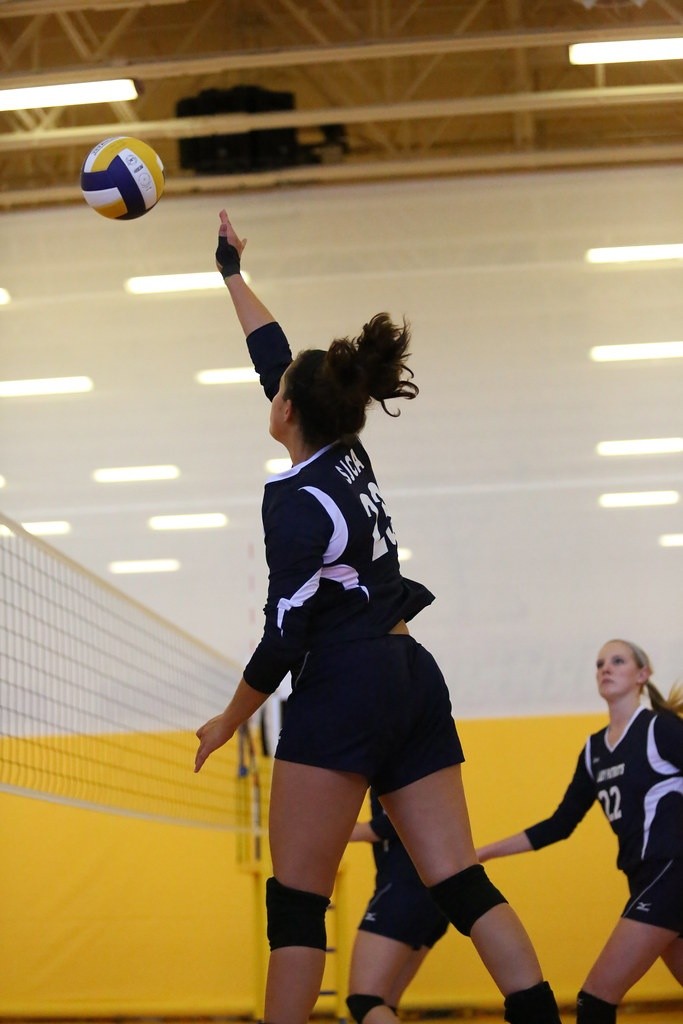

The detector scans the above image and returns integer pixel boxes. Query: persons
[474,639,683,1024]
[195,212,563,1024]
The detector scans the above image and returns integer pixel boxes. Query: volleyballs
[77,133,165,224]
